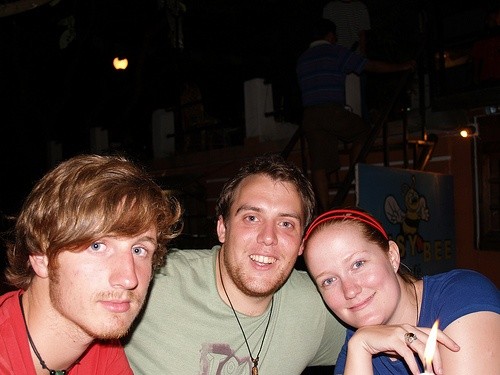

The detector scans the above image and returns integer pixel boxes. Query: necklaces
[19,294,82,375]
[219,249,274,375]
[409,279,418,327]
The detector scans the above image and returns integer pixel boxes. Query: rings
[404,333,417,345]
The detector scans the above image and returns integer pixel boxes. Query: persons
[0,154,184,375]
[303,210,500,375]
[322,0,372,50]
[297,19,417,214]
[424,33,491,130]
[119,156,348,375]
[469,9,500,127]
